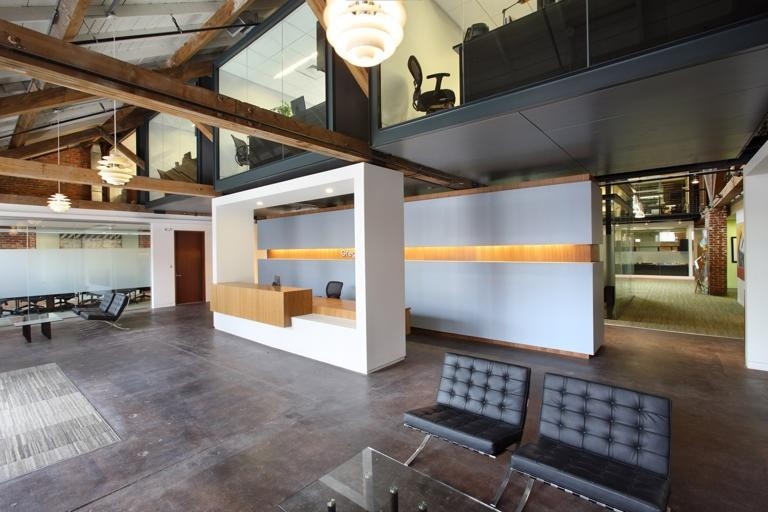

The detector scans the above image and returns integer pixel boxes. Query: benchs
[403,352,532,507]
[511,372,673,511]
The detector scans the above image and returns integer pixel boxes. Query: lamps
[501,0,529,25]
[321,0,406,69]
[46,108,73,214]
[96,11,137,187]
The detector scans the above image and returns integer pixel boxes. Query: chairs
[314,281,343,299]
[72,292,131,332]
[406,55,455,116]
[230,134,250,168]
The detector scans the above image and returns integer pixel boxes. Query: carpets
[1,361,122,485]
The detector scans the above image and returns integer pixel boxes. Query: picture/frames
[731,236,738,264]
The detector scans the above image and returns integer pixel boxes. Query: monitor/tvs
[272,276,280,284]
